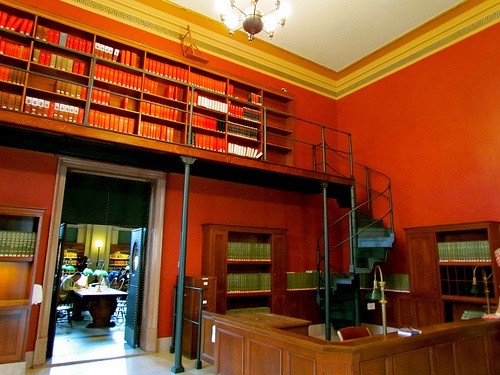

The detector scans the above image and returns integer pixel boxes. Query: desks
[72,283,128,328]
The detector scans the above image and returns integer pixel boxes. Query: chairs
[337,326,372,342]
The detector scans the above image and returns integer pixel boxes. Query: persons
[481,248,500,319]
[57,268,130,322]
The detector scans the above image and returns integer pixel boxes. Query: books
[226,273,272,293]
[0,11,263,160]
[382,273,410,291]
[438,240,493,263]
[287,271,344,289]
[461,309,487,320]
[227,306,271,316]
[227,241,272,262]
[0,230,36,258]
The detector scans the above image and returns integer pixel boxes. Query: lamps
[214,0,293,44]
[62,258,108,292]
[92,240,102,282]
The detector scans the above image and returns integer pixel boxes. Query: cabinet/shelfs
[402,220,500,326]
[201,223,288,315]
[109,244,130,266]
[0,0,294,163]
[63,243,85,274]
